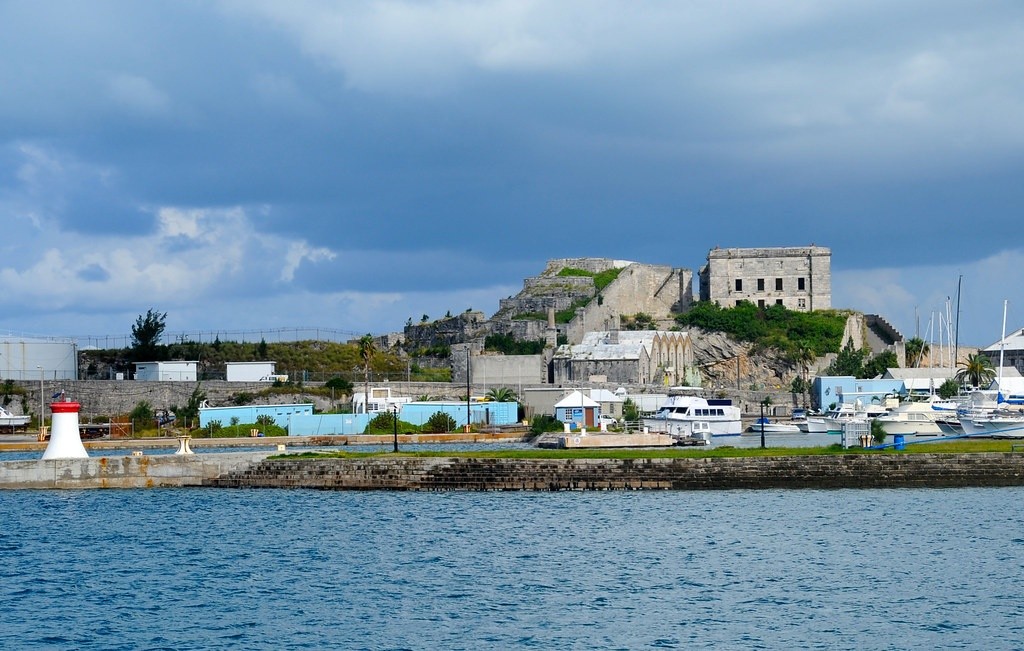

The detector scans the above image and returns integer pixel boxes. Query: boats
[535,273,1024,449]
